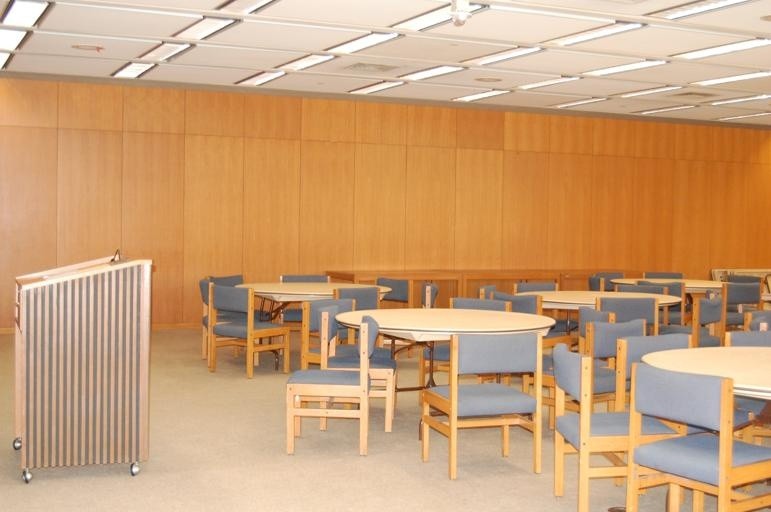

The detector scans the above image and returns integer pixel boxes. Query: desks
[334,308,556,439]
[609,278,725,292]
[515,290,681,335]
[642,345,771,403]
[234,282,392,370]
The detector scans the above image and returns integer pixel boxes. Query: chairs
[725,331,771,346]
[199,279,240,360]
[590,272,623,292]
[660,297,722,348]
[576,306,613,367]
[744,310,771,331]
[625,361,771,512]
[514,282,580,343]
[595,297,658,336]
[552,319,647,439]
[286,323,373,456]
[487,292,573,429]
[209,282,289,378]
[209,276,243,285]
[333,287,381,344]
[420,331,544,479]
[616,333,706,434]
[299,298,355,370]
[551,344,679,512]
[318,305,396,433]
[478,284,494,300]
[375,278,413,356]
[419,297,511,388]
[716,282,760,346]
[738,304,758,329]
[278,275,330,355]
[725,274,763,312]
[420,281,438,308]
[643,272,682,323]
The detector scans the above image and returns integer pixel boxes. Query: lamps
[273,53,336,72]
[549,98,607,109]
[322,31,402,56]
[214,0,276,15]
[691,71,771,88]
[712,94,771,106]
[0,0,52,29]
[0,28,28,51]
[398,66,465,82]
[444,8,473,28]
[620,86,681,99]
[110,63,157,79]
[640,104,695,115]
[349,81,405,96]
[579,59,667,79]
[235,71,286,87]
[72,44,104,52]
[645,1,749,20]
[452,89,511,103]
[540,23,642,49]
[390,2,489,34]
[460,46,542,69]
[132,41,195,64]
[513,77,579,91]
[0,52,13,71]
[713,113,770,121]
[168,14,242,42]
[669,38,771,62]
